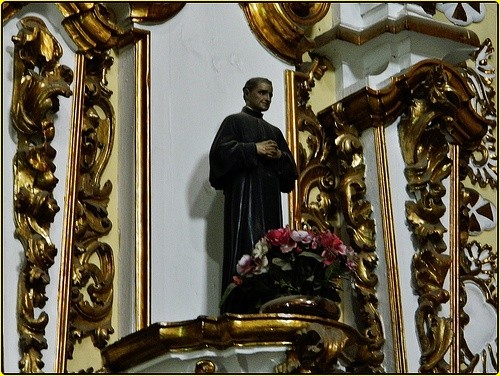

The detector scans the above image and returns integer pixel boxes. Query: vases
[259,294,342,322]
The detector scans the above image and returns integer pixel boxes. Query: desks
[103,311,378,374]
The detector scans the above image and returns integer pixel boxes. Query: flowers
[233,225,361,304]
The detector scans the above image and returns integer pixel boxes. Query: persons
[209,77,298,315]
